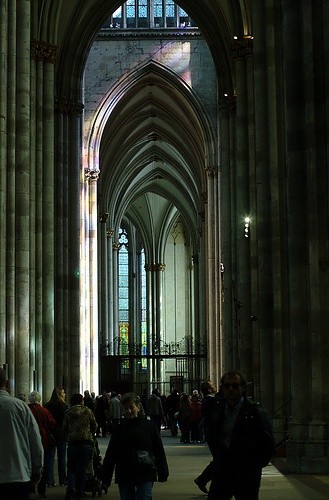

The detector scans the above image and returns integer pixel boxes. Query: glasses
[222,382,239,390]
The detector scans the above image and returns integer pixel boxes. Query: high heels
[60,480,68,487]
[47,481,57,488]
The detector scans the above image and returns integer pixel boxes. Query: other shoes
[179,439,207,445]
[194,477,208,494]
[64,494,82,500]
[96,435,108,438]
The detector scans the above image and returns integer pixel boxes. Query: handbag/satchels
[47,431,57,447]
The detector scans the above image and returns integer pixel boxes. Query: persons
[0,367,269,500]
